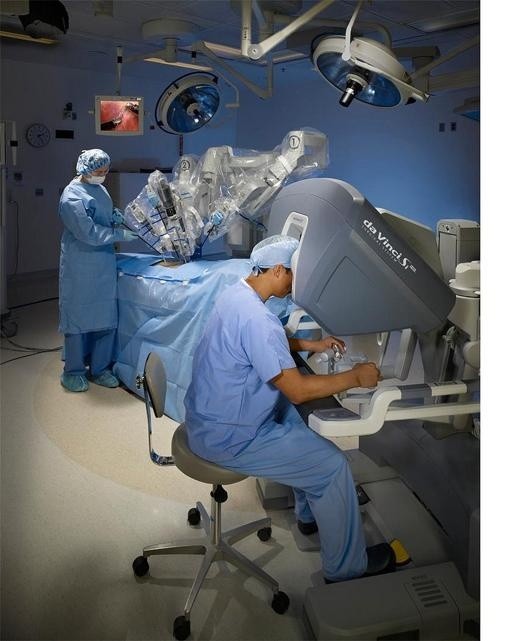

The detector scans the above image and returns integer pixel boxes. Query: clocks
[26,123,51,148]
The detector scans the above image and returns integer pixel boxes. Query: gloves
[112,207,124,224]
[124,229,139,242]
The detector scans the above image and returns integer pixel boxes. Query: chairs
[131,352,291,640]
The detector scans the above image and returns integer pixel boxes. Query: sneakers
[297,520,318,535]
[323,542,395,583]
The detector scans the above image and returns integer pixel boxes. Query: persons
[59,147,140,392]
[181,235,396,586]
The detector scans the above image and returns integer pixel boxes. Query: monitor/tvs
[95,95,144,136]
